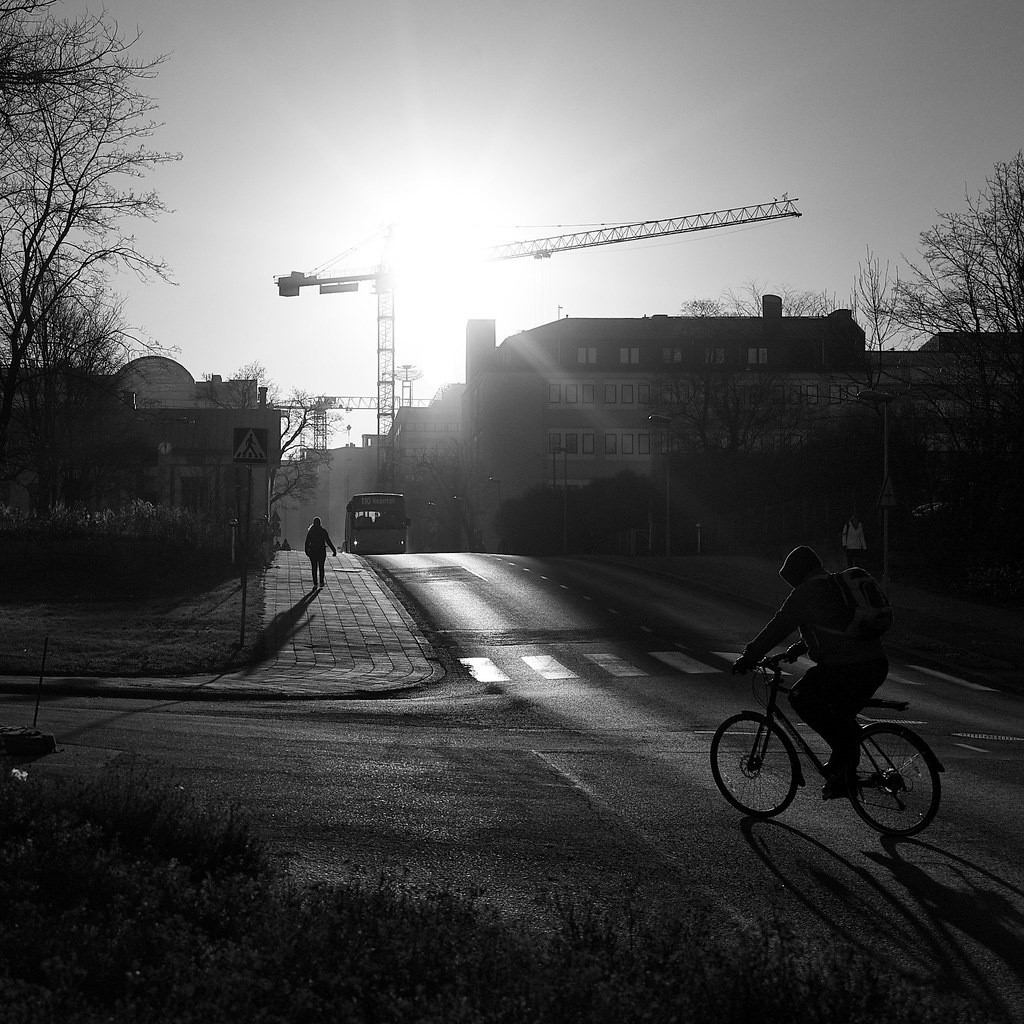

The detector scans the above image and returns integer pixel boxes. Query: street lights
[553,445,569,558]
[648,414,673,555]
[490,475,503,555]
[453,495,465,552]
[858,387,897,605]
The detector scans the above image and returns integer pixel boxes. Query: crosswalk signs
[232,427,267,466]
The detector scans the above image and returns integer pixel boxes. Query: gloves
[729,661,746,676]
[780,642,800,664]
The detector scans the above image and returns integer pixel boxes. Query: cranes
[258,396,438,449]
[275,192,802,493]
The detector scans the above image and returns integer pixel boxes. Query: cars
[338,541,346,553]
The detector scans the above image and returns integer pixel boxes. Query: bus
[345,494,412,555]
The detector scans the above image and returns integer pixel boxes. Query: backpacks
[801,566,896,646]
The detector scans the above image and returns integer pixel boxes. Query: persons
[275,539,290,551]
[305,517,336,586]
[733,545,888,798]
[842,516,867,568]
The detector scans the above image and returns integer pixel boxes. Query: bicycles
[710,652,944,839]
